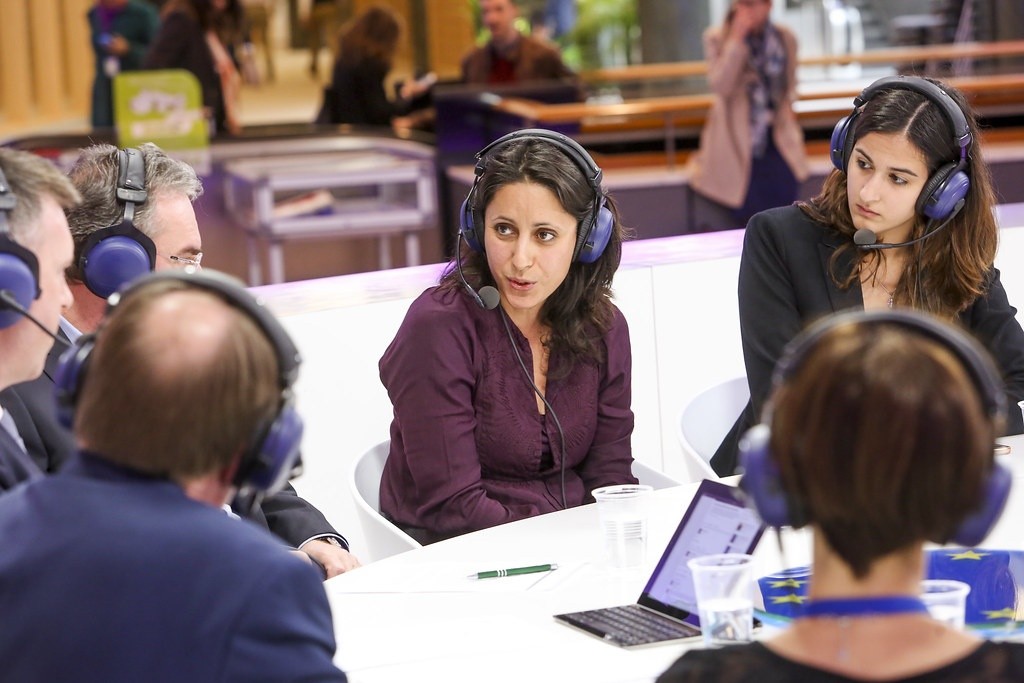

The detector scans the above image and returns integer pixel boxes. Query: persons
[88,0,163,130]
[378,129,638,548]
[326,7,426,124]
[0,143,362,581]
[0,148,82,492]
[687,0,812,228]
[0,265,347,683]
[650,307,1024,683]
[736,76,1024,438]
[459,0,563,84]
[153,0,244,142]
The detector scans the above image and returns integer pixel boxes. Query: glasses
[156,251,202,273]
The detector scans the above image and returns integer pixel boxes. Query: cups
[686,553,758,648]
[1018,401,1024,422]
[592,484,655,572]
[920,579,972,634]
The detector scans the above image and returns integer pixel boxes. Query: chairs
[677,377,752,482]
[350,439,683,564]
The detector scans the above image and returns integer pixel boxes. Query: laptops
[552,479,768,650]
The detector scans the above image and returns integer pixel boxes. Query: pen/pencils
[467,563,558,580]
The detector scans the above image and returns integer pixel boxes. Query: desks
[238,203,1024,564]
[318,435,1023,683]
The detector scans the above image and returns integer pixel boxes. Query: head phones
[0,167,42,331]
[78,147,157,299]
[740,304,1013,548]
[459,128,613,263]
[53,264,305,497]
[830,74,973,219]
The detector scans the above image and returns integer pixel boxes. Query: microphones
[458,231,500,310]
[853,198,965,251]
